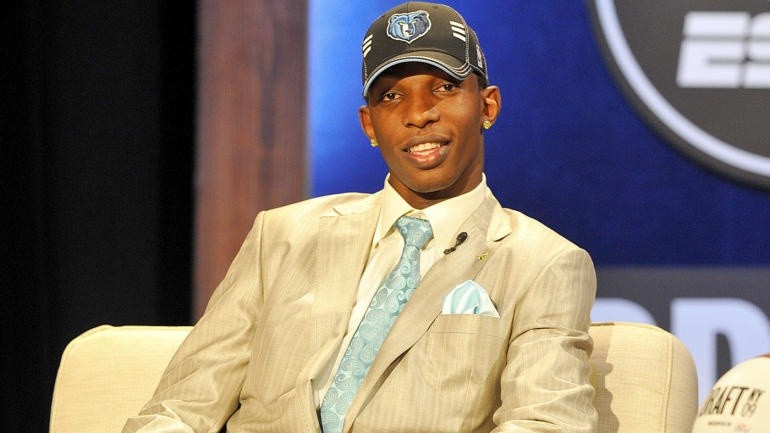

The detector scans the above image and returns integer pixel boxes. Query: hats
[362,1,492,96]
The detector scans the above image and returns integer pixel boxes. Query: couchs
[48,320,700,432]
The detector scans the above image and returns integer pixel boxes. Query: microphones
[444,231,467,256]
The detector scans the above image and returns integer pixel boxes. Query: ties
[320,217,432,433]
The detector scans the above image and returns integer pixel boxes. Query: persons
[116,2,603,433]
